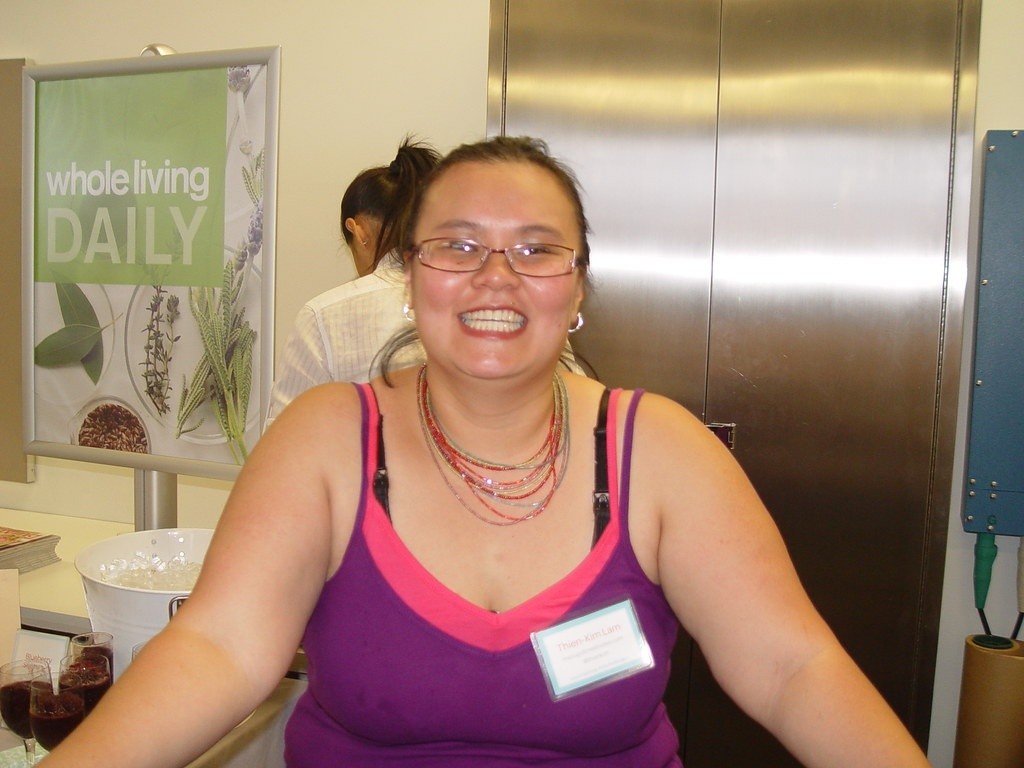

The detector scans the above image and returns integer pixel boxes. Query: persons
[261,144,586,437]
[24,139,935,768]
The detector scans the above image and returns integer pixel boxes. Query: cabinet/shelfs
[487,0,981,768]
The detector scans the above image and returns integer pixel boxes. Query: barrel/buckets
[73,526,258,729]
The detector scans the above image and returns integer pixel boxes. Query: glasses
[409,237,582,278]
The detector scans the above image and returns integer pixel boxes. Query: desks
[1,560,312,672]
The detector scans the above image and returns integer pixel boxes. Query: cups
[68,632,114,684]
[29,671,86,753]
[58,653,111,717]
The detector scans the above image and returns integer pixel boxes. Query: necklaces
[416,360,571,526]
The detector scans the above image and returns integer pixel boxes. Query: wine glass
[0,658,53,768]
